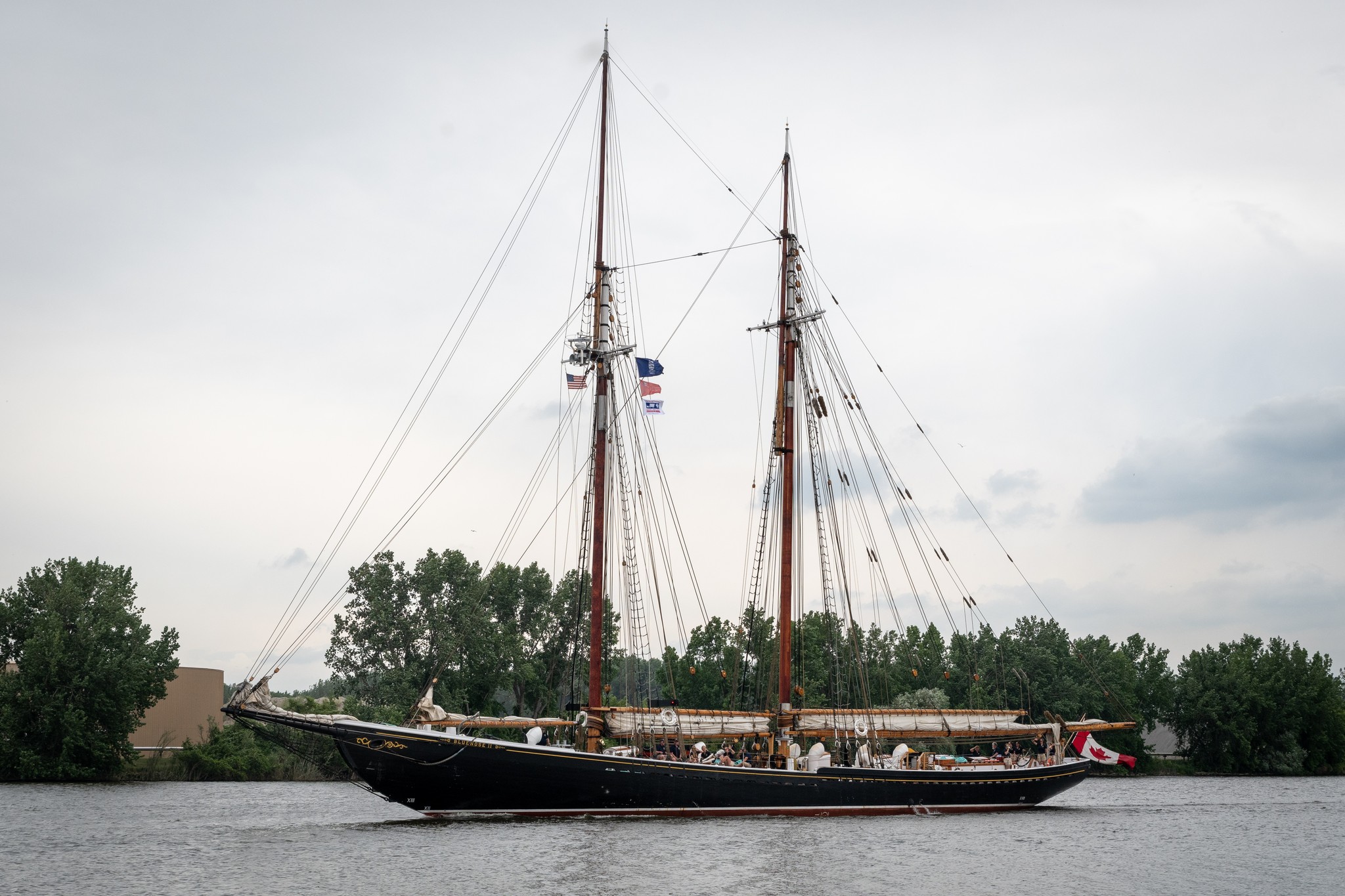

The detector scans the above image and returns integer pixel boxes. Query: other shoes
[713,763,719,765]
[691,761,696,763]
[719,763,725,766]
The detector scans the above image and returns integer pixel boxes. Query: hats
[741,747,745,750]
[660,739,664,742]
[674,739,678,741]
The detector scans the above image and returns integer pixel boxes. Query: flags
[566,373,587,389]
[638,379,662,397]
[641,398,664,415]
[1072,724,1138,768]
[634,356,664,378]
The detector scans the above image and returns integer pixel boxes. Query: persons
[528,720,1066,776]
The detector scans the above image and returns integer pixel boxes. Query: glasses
[1006,745,1009,747]
[675,741,678,742]
[724,748,728,750]
[1016,743,1019,745]
[661,741,665,743]
[541,729,544,730]
[742,749,745,751]
[1040,741,1043,742]
[1009,744,1011,745]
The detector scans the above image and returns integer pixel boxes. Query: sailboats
[217,20,1144,825]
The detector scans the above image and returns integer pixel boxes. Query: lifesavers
[576,710,588,728]
[660,708,677,726]
